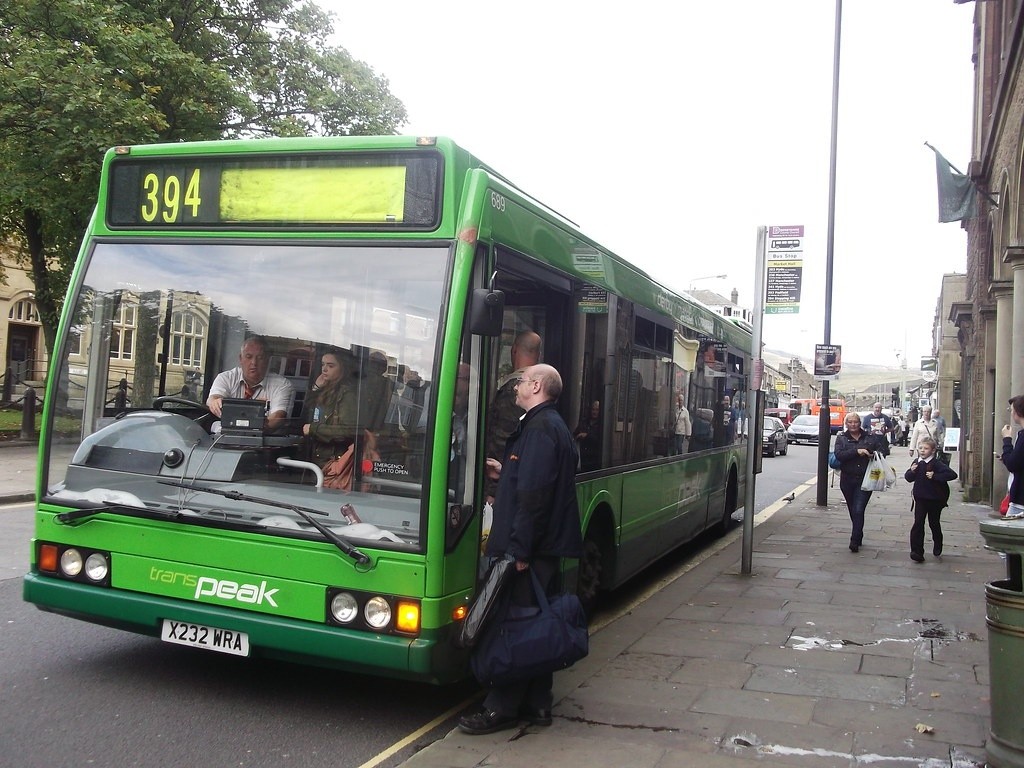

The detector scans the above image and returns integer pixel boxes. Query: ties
[243,383,259,400]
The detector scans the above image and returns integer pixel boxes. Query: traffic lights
[891,386,899,404]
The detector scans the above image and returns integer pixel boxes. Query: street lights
[689,274,727,295]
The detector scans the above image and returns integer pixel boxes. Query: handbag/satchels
[459,559,511,649]
[469,561,589,679]
[861,451,896,492]
[999,496,1011,515]
[829,454,840,470]
[935,447,946,464]
[321,443,381,495]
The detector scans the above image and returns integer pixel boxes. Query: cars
[787,414,832,444]
[763,416,788,458]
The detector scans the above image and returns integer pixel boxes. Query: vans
[764,407,798,430]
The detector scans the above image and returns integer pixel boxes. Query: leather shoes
[458,705,521,734]
[519,704,552,726]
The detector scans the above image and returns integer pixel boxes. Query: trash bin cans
[978,517,1024,768]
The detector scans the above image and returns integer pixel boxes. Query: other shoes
[909,552,925,562]
[933,539,944,556]
[849,545,858,552]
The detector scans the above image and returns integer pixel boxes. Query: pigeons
[782,493,795,503]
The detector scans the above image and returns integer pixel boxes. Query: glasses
[515,378,537,386]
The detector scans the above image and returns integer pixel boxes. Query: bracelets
[312,382,320,390]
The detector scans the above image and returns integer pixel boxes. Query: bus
[787,397,847,436]
[20,134,766,686]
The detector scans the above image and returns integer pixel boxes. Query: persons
[570,400,599,465]
[457,363,587,735]
[484,330,542,506]
[206,336,295,452]
[447,361,479,462]
[909,405,946,458]
[861,402,894,455]
[993,393,1024,559]
[669,395,746,503]
[302,350,358,482]
[904,437,958,562]
[891,415,910,447]
[834,412,886,553]
